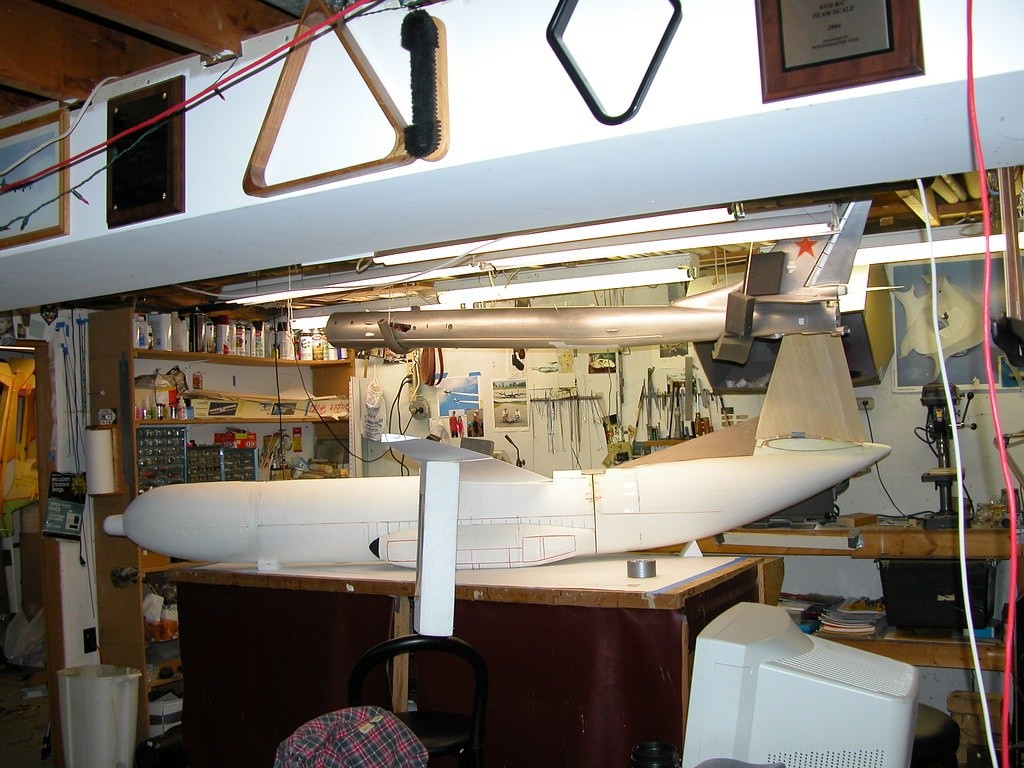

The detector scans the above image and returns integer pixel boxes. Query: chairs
[343,632,490,768]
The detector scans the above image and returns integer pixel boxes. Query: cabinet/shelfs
[724,521,1016,684]
[84,310,353,748]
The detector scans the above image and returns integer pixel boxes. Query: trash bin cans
[56,663,142,768]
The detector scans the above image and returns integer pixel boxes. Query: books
[775,593,886,638]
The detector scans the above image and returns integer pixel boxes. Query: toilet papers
[84,429,115,495]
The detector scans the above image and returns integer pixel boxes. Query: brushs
[401,8,448,162]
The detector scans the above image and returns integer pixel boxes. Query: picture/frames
[882,249,1023,394]
[0,109,70,251]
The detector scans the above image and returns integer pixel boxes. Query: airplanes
[499,386,526,398]
[325,201,870,348]
[453,398,460,404]
[443,389,452,394]
[103,332,894,570]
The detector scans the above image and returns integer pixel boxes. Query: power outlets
[855,397,875,410]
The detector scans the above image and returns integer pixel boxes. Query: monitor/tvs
[682,601,920,768]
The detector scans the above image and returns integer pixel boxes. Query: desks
[136,553,760,768]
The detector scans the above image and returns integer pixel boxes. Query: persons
[468,410,483,436]
[501,408,521,422]
[450,411,464,438]
